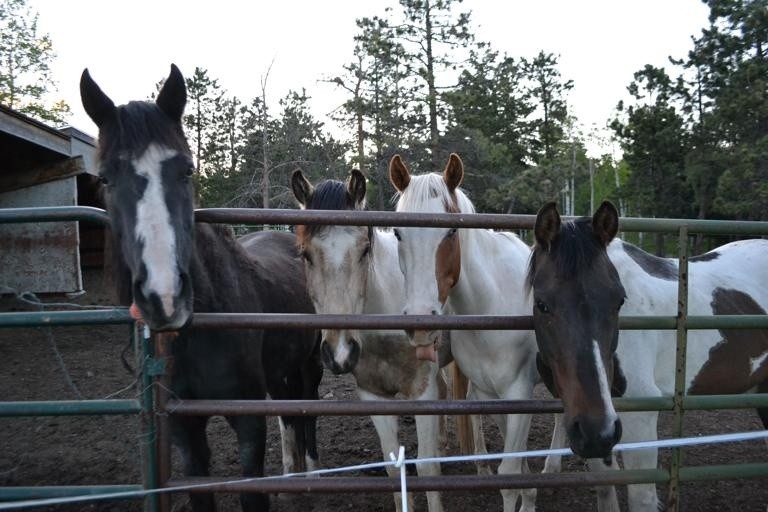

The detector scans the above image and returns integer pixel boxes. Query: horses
[387,151,568,511]
[288,164,495,511]
[522,199,768,512]
[78,63,325,512]
